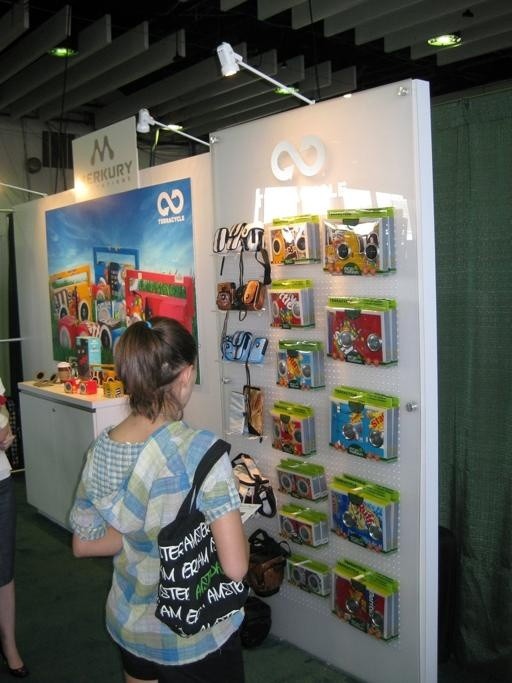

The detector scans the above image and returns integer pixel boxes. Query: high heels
[0,641,28,677]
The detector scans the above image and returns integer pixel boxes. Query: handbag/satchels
[247,528,292,596]
[155,438,250,638]
[231,452,277,517]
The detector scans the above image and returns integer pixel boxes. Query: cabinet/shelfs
[17,379,145,538]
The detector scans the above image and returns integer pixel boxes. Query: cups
[57,362,70,383]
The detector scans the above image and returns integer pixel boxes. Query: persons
[70,314,259,683]
[0,380,33,678]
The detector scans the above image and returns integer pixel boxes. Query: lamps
[215,40,316,105]
[137,105,210,148]
